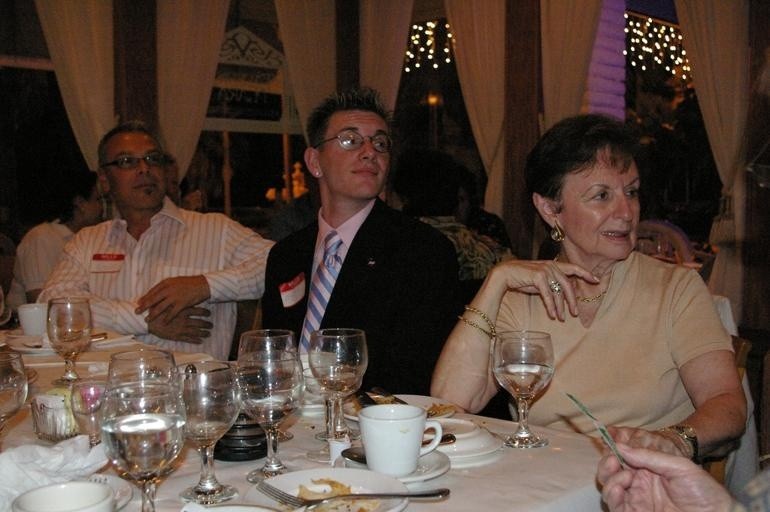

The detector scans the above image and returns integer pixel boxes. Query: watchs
[666,423,698,460]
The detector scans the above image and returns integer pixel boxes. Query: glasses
[314,129,393,153]
[101,154,163,168]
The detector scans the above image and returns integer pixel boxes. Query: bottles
[13,479,115,512]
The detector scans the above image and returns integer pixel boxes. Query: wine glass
[107,349,177,383]
[238,329,296,359]
[237,349,306,483]
[71,376,120,452]
[0,352,29,423]
[308,328,369,441]
[46,297,94,387]
[492,329,555,448]
[169,360,243,507]
[98,384,189,512]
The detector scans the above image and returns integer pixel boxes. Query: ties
[297,232,342,354]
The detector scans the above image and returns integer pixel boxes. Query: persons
[431,112,752,462]
[159,153,208,212]
[263,86,461,396]
[29,120,278,362]
[595,441,749,511]
[6,169,106,314]
[266,145,515,290]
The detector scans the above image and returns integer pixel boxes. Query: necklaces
[572,292,607,302]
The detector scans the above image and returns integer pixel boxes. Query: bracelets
[457,303,497,338]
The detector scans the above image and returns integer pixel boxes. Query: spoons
[338,432,457,464]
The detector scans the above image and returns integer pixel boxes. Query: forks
[254,480,453,509]
[21,332,108,349]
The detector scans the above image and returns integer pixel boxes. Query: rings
[549,281,559,292]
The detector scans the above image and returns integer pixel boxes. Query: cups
[357,402,443,477]
[17,303,47,336]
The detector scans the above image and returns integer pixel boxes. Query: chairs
[698,332,754,488]
[226,297,266,362]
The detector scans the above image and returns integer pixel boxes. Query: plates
[340,394,503,460]
[334,446,449,481]
[86,473,134,510]
[6,337,57,355]
[243,467,408,511]
